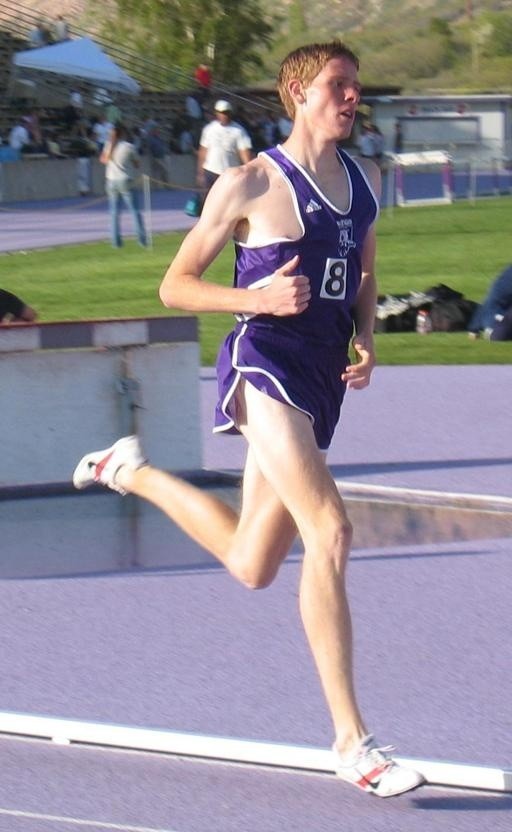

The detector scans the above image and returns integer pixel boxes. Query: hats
[214,99,232,114]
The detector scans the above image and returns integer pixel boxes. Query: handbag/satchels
[184,190,200,219]
[373,282,484,335]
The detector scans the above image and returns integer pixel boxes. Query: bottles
[416,310,432,333]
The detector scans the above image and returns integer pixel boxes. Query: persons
[354,120,388,176]
[7,106,68,158]
[192,61,213,87]
[395,122,403,154]
[29,17,68,46]
[72,44,427,799]
[100,125,148,248]
[71,88,212,158]
[467,266,511,342]
[234,107,293,152]
[195,100,254,221]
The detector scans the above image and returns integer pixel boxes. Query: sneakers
[113,241,149,249]
[326,732,427,799]
[69,433,147,498]
